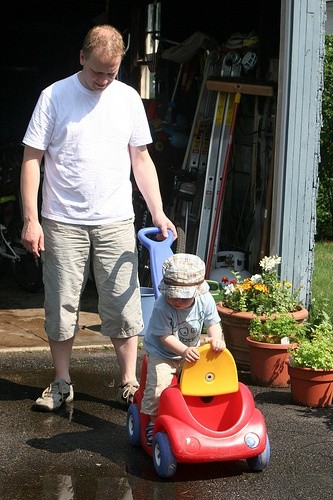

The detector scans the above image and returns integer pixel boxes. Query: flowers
[217,253,295,313]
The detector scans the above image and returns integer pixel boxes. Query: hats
[158,254,211,299]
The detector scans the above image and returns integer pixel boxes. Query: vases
[216,305,308,380]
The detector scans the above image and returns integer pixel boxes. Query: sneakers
[116,381,140,407]
[35,377,74,410]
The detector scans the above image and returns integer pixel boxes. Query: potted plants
[289,297,333,406]
[244,312,309,389]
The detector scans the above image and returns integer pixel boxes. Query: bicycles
[0,195,43,293]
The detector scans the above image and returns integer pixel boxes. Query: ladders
[170,51,242,271]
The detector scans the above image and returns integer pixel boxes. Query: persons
[140,253,226,446]
[20,25,178,411]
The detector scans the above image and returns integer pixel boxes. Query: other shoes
[145,423,154,445]
